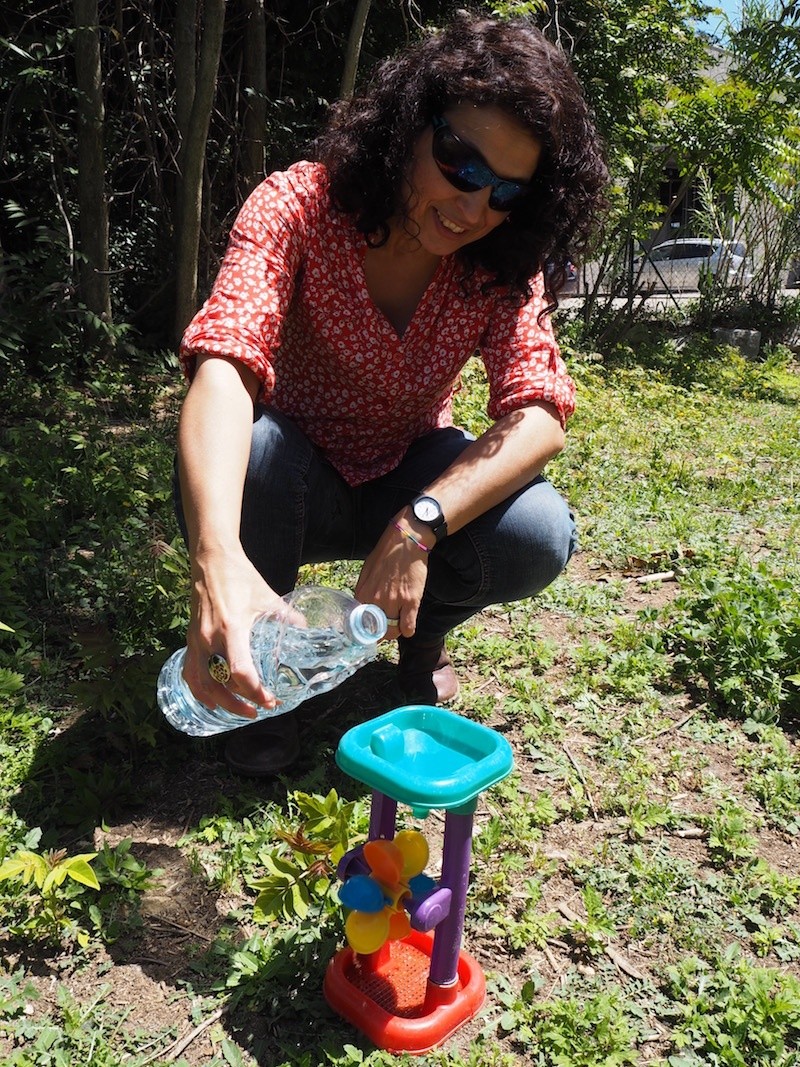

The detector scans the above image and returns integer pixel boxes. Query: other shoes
[399,635,460,708]
[214,714,303,779]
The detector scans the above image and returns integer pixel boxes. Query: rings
[207,654,231,684]
[385,617,401,625]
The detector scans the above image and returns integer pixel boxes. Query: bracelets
[389,518,432,553]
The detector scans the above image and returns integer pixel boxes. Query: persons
[172,15,615,768]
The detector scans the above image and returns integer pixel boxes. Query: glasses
[431,114,538,213]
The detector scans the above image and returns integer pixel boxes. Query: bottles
[157,586,388,737]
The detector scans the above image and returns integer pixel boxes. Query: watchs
[408,490,448,542]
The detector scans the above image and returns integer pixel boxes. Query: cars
[543,253,578,299]
[614,238,751,299]
